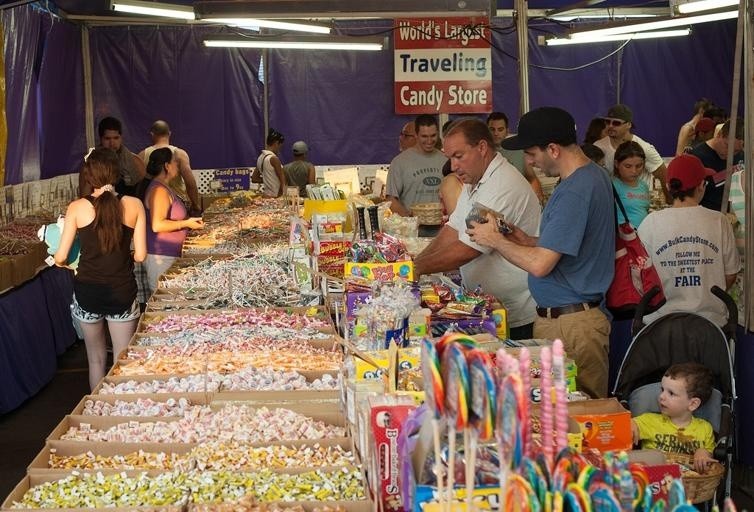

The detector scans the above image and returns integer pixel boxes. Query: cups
[608,119,629,126]
[399,131,416,138]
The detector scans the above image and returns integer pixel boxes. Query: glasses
[202,35,384,52]
[565,8,739,41]
[544,25,694,47]
[109,0,201,21]
[669,0,739,17]
[203,17,334,35]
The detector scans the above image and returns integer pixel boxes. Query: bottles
[665,152,715,193]
[292,140,312,155]
[688,118,715,137]
[500,106,576,151]
[607,104,638,130]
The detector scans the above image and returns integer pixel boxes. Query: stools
[535,301,601,319]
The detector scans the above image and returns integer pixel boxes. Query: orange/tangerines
[607,284,740,512]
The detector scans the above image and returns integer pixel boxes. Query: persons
[79,116,145,200]
[585,117,607,144]
[594,105,674,206]
[54,147,147,393]
[612,142,651,229]
[386,117,450,217]
[468,107,617,399]
[675,99,713,156]
[398,113,523,227]
[145,147,205,294]
[415,117,542,340]
[282,141,315,196]
[630,361,717,471]
[691,117,744,213]
[137,120,202,217]
[637,154,743,332]
[487,112,541,199]
[252,128,286,197]
[689,118,716,145]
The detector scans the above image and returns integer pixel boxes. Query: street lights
[606,178,666,319]
[252,154,270,183]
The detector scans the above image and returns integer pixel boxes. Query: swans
[724,496,736,512]
[108,305,343,376]
[506,445,698,512]
[96,364,339,395]
[60,397,345,443]
[420,330,527,469]
